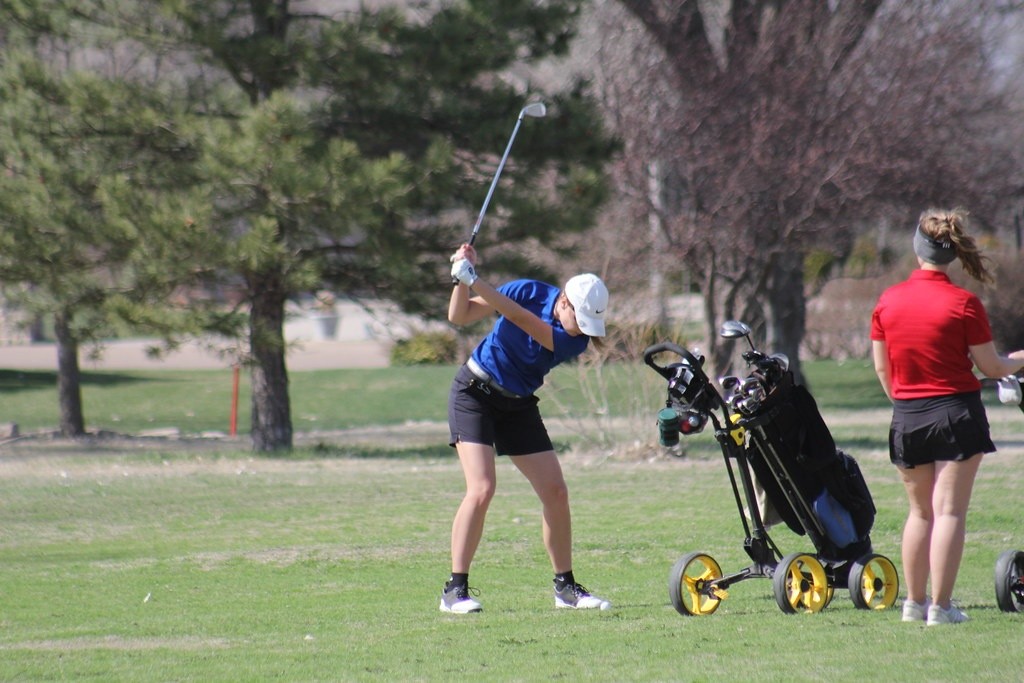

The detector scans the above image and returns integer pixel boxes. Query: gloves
[450,259,478,288]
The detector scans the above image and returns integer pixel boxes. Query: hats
[564,273,610,338]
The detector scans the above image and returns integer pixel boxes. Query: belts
[465,356,525,400]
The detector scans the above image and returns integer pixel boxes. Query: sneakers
[438,588,482,614]
[553,585,610,610]
[926,605,969,626]
[901,596,932,622]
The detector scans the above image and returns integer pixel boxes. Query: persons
[869,207,1024,625]
[443,243,615,612]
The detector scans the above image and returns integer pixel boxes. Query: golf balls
[600,601,611,611]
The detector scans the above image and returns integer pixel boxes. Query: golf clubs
[452,103,547,286]
[718,320,783,417]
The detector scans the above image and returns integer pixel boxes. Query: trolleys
[644,340,902,618]
